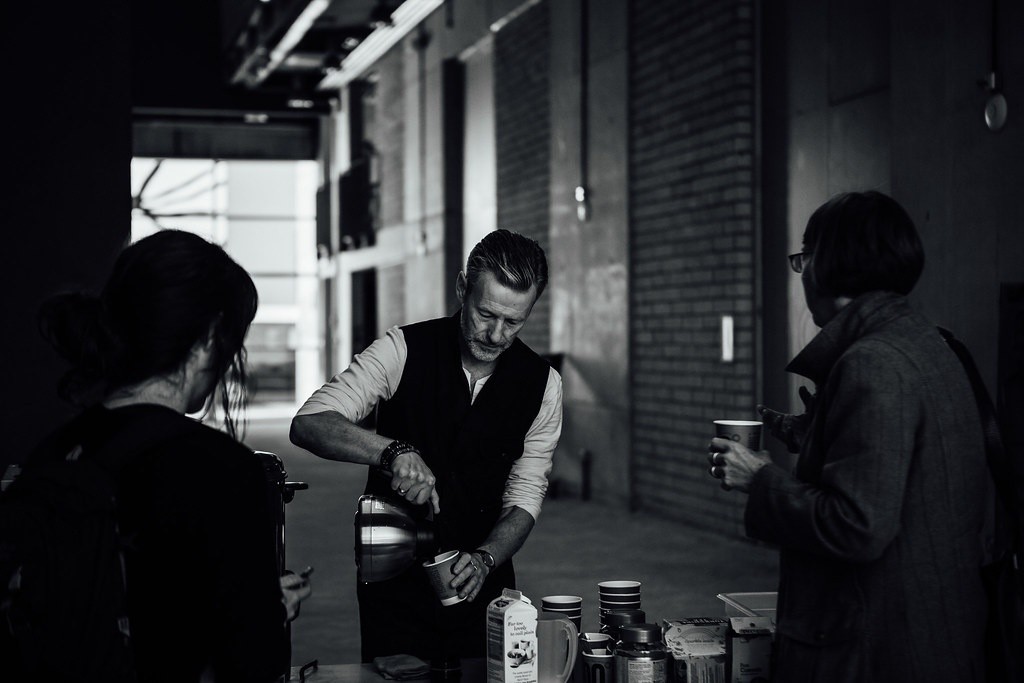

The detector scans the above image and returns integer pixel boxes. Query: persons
[0,229,313,683]
[289,229,565,668]
[708,191,1024,683]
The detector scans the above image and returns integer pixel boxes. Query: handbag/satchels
[981,554,1024,683]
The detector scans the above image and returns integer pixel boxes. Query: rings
[467,561,478,572]
[398,487,407,494]
[711,466,718,478]
[712,453,719,465]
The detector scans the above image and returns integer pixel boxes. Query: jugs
[355,488,441,584]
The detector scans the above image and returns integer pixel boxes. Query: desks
[285,652,487,683]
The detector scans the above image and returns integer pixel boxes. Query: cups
[599,580,642,635]
[422,549,469,606]
[541,595,582,609]
[713,418,764,456]
[578,632,611,650]
[540,606,582,617]
[582,649,615,683]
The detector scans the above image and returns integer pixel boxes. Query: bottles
[568,614,582,633]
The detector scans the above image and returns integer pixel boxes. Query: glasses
[787,251,813,273]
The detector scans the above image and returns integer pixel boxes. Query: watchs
[472,549,496,572]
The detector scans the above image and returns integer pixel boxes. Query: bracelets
[379,439,421,472]
